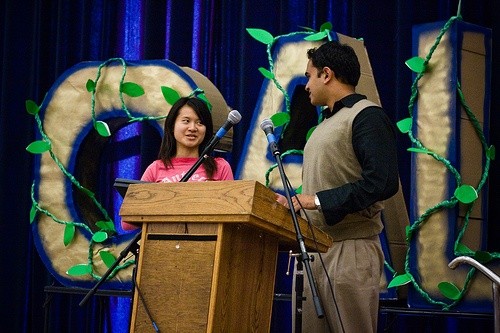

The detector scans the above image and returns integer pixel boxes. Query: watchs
[314,194,322,213]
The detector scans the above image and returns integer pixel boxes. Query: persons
[121,97,234,231]
[275,41,400,333]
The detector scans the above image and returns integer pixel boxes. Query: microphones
[203,110,242,158]
[260,118,280,157]
